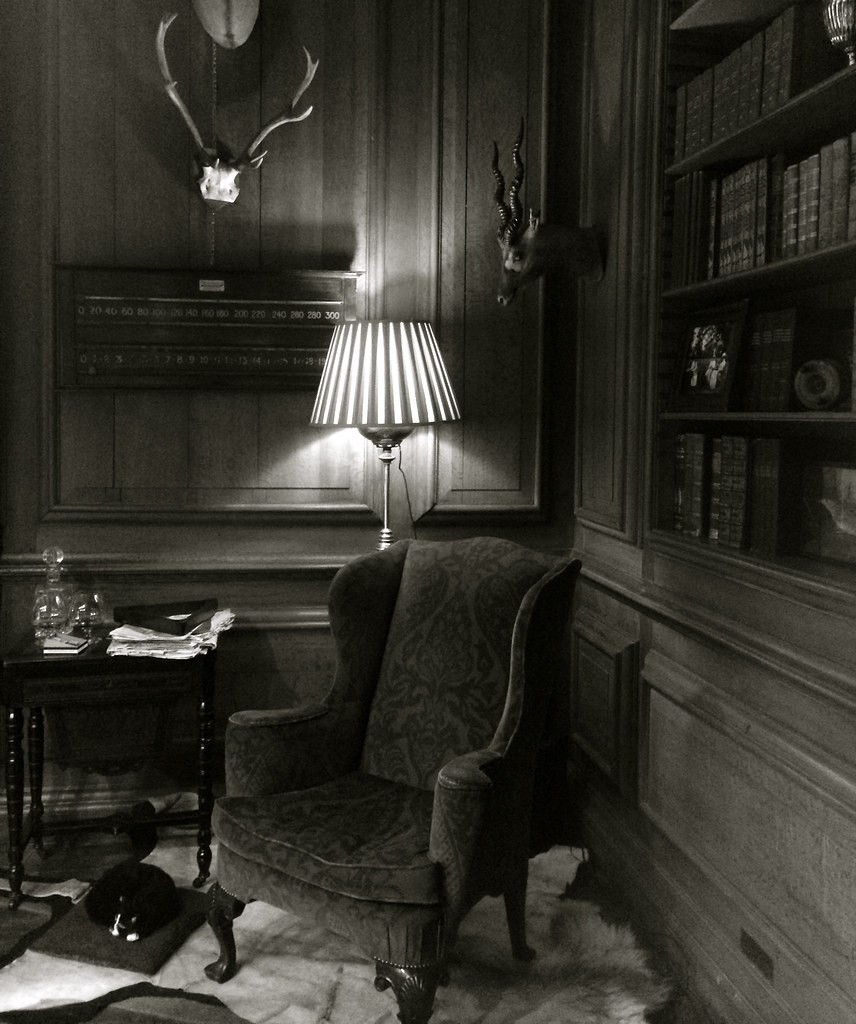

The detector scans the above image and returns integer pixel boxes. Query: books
[659,5,856,554]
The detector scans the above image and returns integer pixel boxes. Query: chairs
[206,535,582,1024]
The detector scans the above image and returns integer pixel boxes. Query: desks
[0,598,218,913]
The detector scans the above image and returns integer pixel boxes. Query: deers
[490,115,606,305]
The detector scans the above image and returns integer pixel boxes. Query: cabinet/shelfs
[652,0,856,592]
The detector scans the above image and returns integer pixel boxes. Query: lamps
[306,319,459,555]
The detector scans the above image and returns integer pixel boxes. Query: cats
[82,858,179,942]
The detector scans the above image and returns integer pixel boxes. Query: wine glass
[68,591,108,648]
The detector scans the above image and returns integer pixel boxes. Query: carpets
[0,830,674,1024]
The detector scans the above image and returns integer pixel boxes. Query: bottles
[29,546,75,637]
